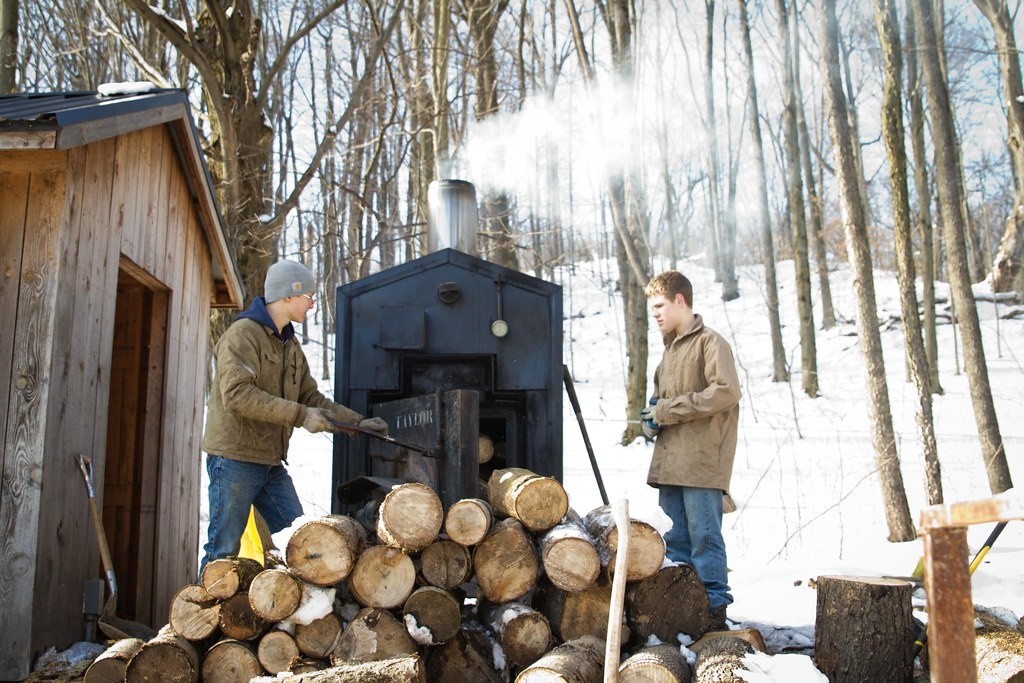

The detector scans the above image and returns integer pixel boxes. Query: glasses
[302,293,317,305]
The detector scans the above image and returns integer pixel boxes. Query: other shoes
[708,604,730,630]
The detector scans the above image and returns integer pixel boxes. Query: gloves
[358,416,389,437]
[640,414,660,439]
[301,406,340,433]
[640,398,661,428]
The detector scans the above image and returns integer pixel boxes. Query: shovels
[562,360,610,505]
[74,451,159,643]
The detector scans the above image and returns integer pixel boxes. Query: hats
[263,259,316,303]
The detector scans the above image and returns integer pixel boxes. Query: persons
[644,270,743,624]
[196,259,388,587]
